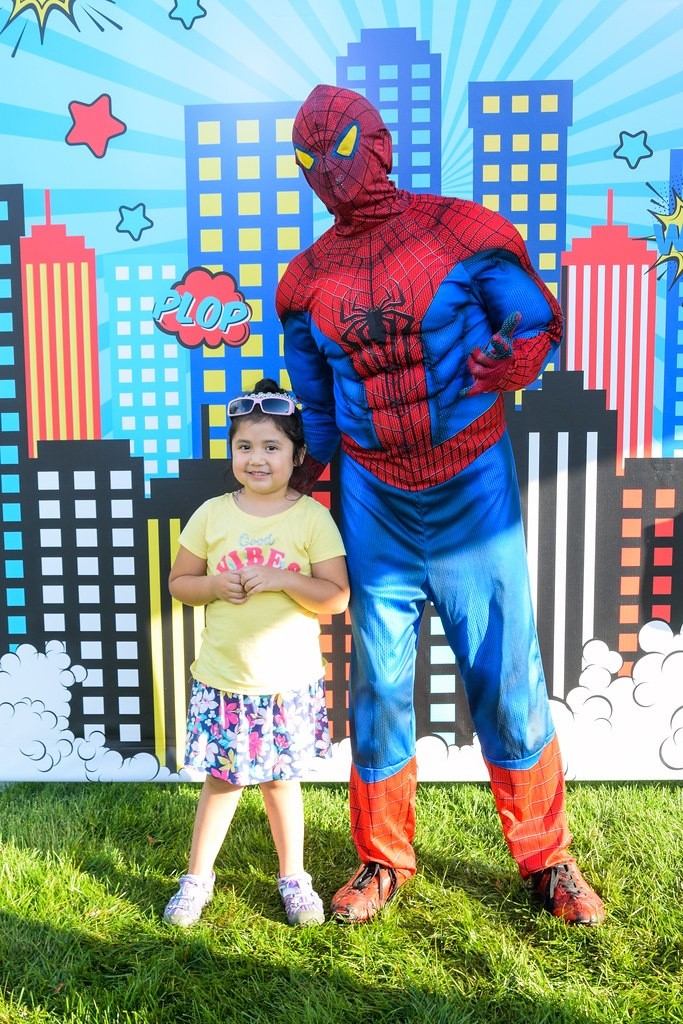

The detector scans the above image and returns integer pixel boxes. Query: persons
[273,84,604,922]
[163,379,350,926]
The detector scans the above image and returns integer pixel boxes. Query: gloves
[458,312,522,400]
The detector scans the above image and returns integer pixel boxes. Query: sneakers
[163,872,217,928]
[531,859,605,927]
[330,857,406,922]
[278,872,321,930]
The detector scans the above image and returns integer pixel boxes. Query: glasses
[227,393,298,423]
